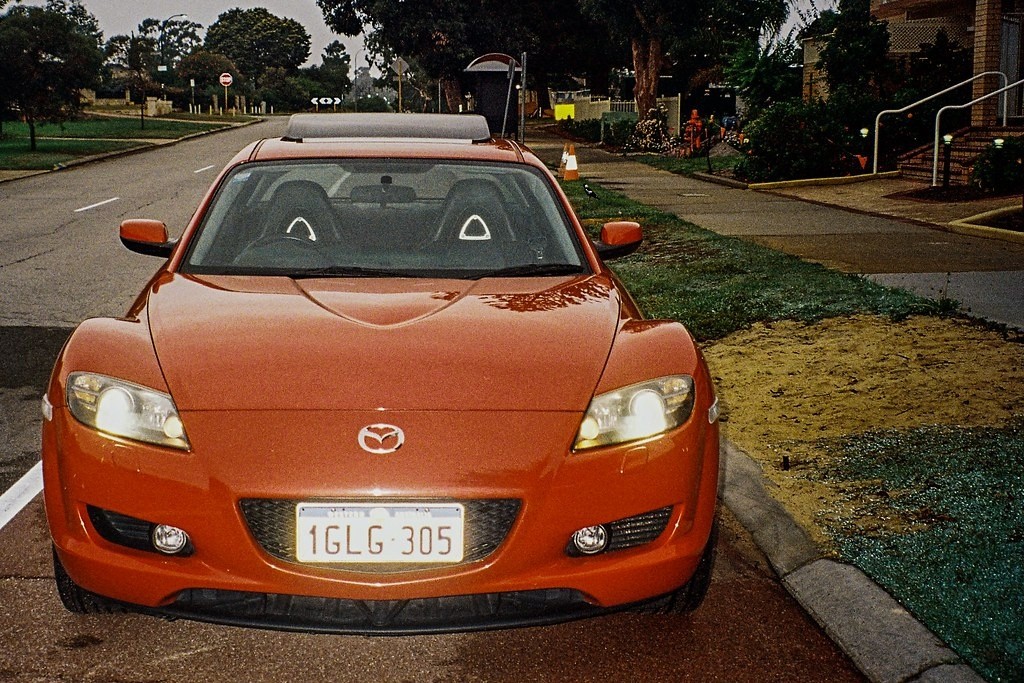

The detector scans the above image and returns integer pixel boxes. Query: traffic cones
[564,144,579,181]
[559,143,569,178]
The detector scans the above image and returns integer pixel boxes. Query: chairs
[415,177,524,253]
[254,181,353,249]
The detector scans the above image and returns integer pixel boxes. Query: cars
[41,114,725,633]
